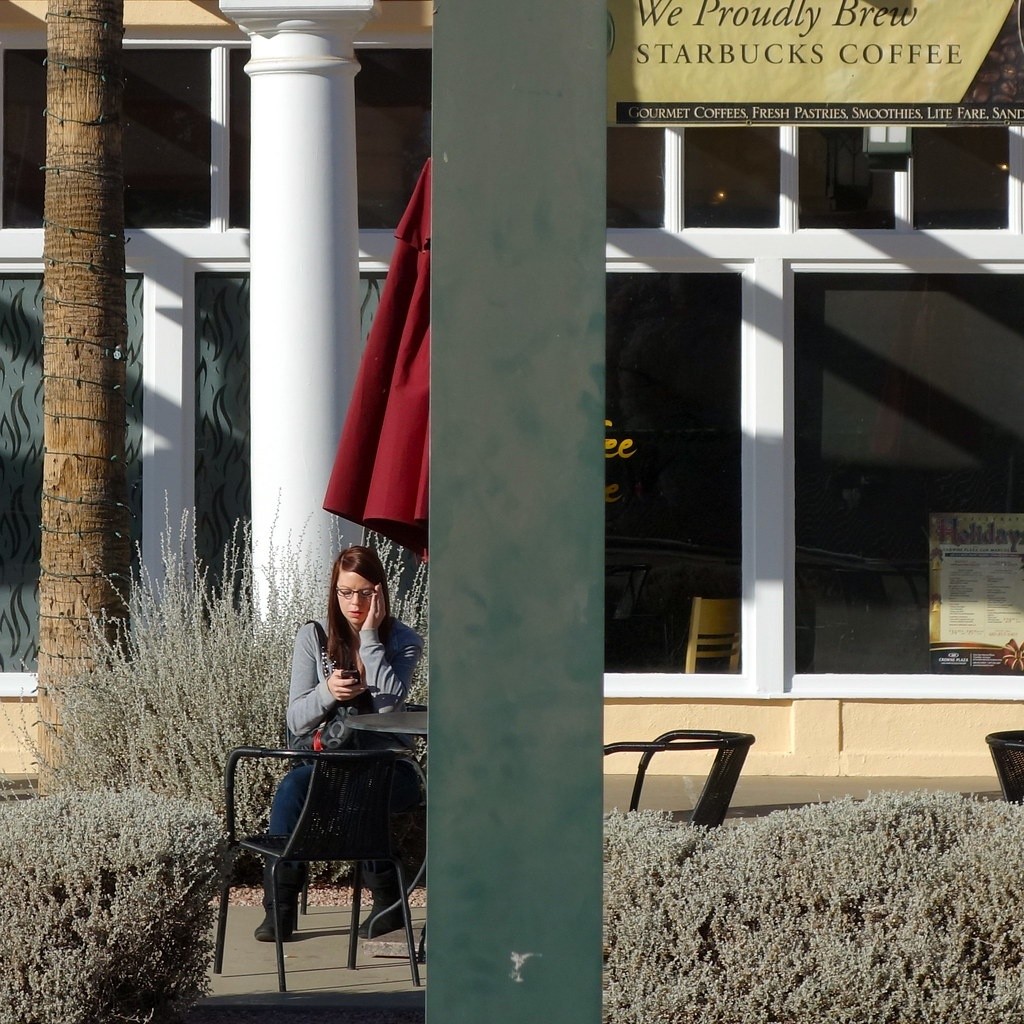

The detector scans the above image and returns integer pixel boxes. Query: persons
[253,544,424,943]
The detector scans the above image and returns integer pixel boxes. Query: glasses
[336,588,375,598]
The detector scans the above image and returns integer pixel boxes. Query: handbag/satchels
[288,620,374,770]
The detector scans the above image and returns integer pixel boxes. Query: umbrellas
[320,156,431,566]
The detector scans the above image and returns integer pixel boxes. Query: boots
[358,867,411,938]
[255,858,298,942]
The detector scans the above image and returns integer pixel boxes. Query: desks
[346,711,427,963]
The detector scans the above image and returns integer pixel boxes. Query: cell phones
[341,670,360,686]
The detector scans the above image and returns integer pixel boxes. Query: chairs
[685,596,744,674]
[985,729,1024,805]
[285,706,429,928]
[605,563,653,654]
[603,729,755,835]
[213,748,426,993]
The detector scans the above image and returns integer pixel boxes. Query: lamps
[821,128,876,213]
